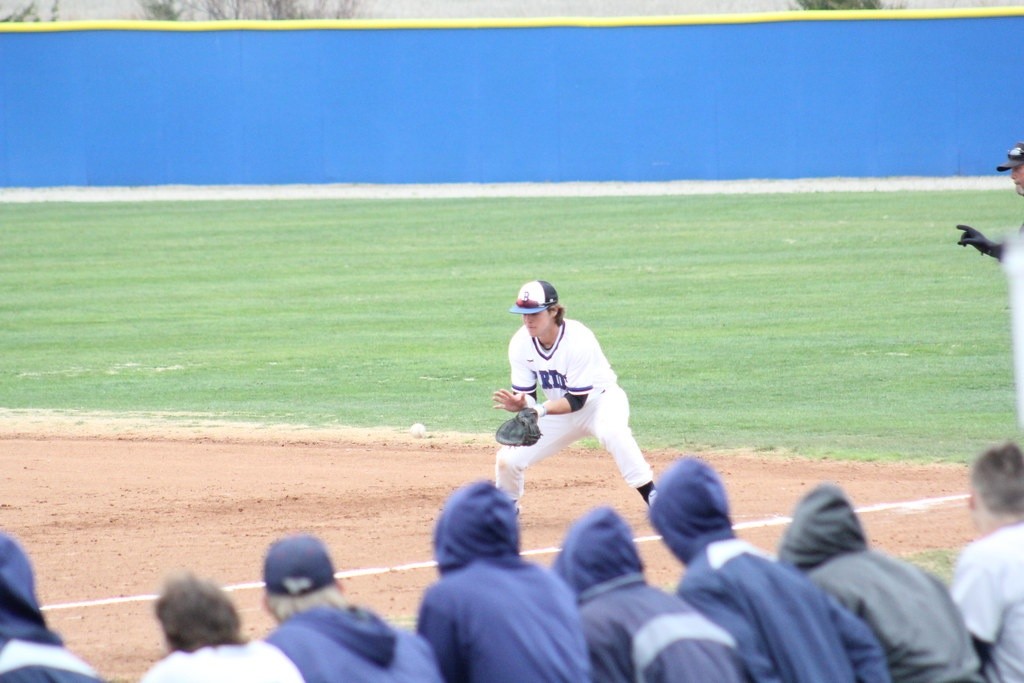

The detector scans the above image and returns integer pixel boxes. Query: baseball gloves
[494,407,544,449]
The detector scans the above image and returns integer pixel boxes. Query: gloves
[956,225,1001,258]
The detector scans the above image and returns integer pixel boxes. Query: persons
[549,506,747,683]
[0,532,107,683]
[136,569,303,683]
[493,279,658,518]
[774,483,978,683]
[258,532,444,683]
[415,479,589,683]
[649,456,891,683]
[956,143,1024,267]
[949,442,1024,681]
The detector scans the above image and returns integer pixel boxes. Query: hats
[264,536,336,596]
[509,280,558,314]
[996,142,1024,171]
